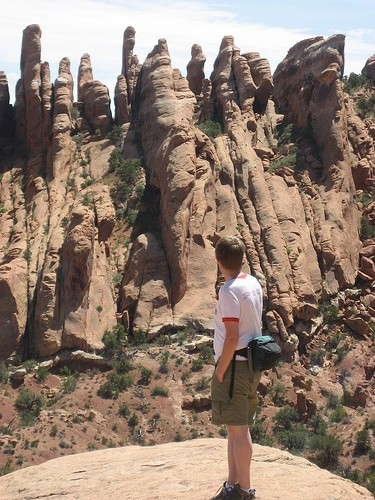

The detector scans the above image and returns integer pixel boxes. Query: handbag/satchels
[235,336,282,373]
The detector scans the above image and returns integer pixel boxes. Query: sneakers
[210,481,230,500]
[222,484,257,500]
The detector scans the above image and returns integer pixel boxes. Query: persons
[210,235,264,500]
